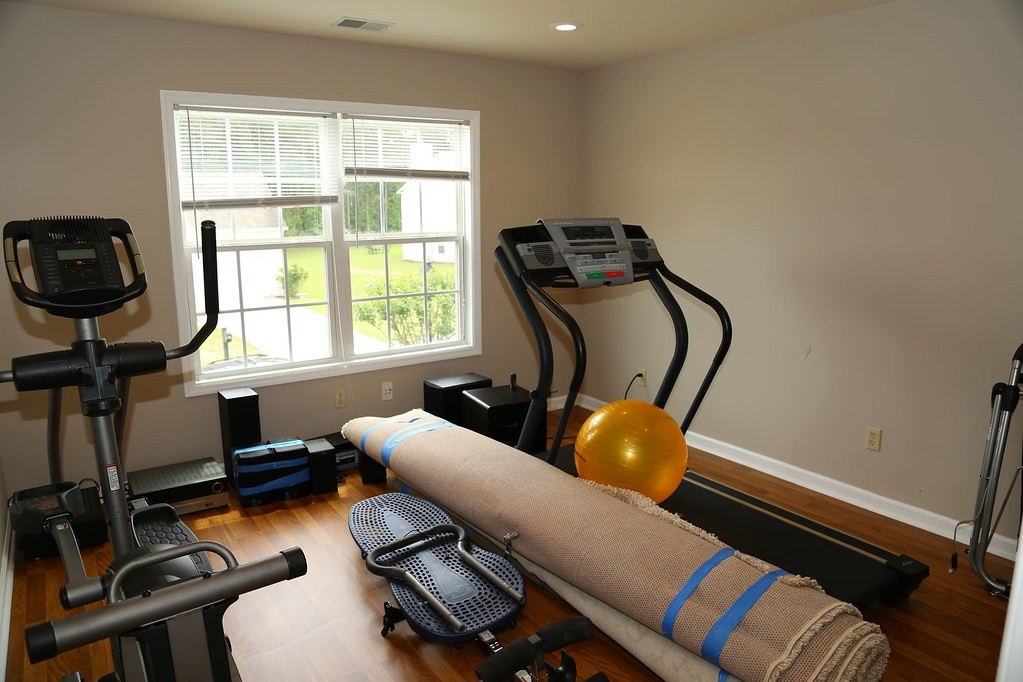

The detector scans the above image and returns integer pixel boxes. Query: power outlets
[864,425,883,452]
[334,387,347,409]
[636,367,647,387]
[381,381,394,402]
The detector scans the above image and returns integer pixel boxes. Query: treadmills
[493,216,929,617]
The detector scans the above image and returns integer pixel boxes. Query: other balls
[573,399,689,505]
